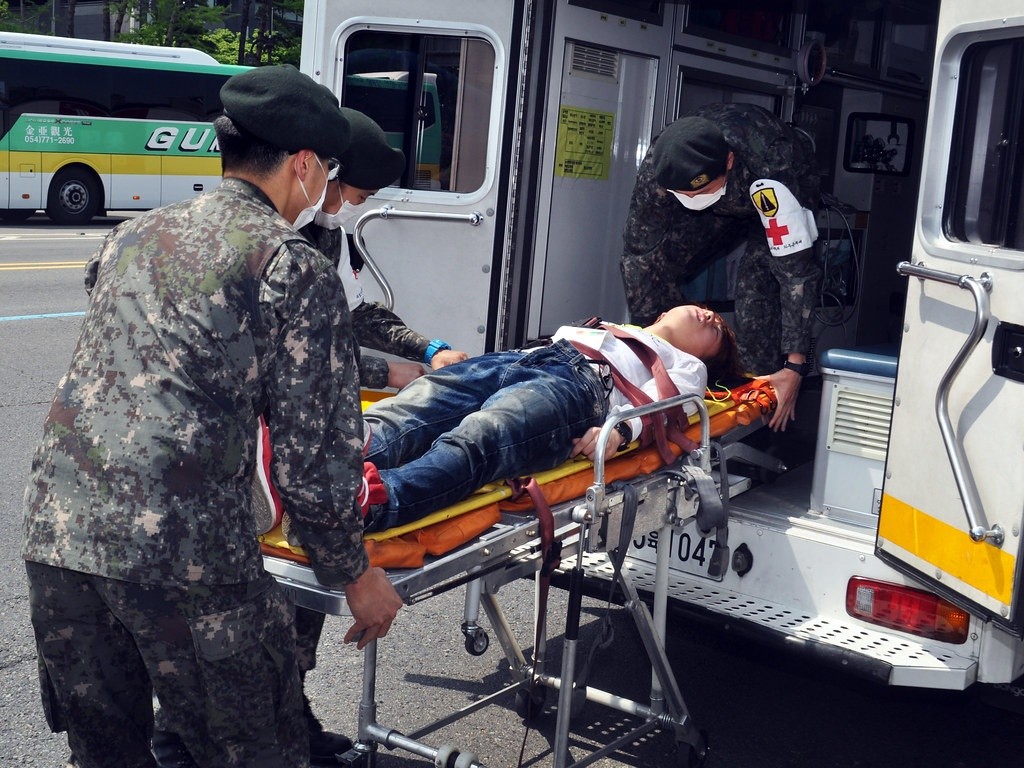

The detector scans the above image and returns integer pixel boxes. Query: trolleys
[259,437,750,767]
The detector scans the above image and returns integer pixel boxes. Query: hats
[334,107,407,191]
[652,116,729,192]
[220,64,351,157]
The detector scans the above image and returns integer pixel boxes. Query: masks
[665,173,729,212]
[315,175,366,231]
[291,152,329,233]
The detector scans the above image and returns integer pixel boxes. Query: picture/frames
[843,112,916,178]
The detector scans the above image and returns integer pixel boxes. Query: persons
[253,303,741,548]
[620,103,822,484]
[22,64,468,768]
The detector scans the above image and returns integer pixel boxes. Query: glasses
[326,155,342,181]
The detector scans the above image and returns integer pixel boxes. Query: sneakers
[356,418,388,521]
[249,410,283,534]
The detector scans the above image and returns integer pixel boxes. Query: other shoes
[302,692,354,766]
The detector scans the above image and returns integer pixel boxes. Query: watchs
[425,338,451,366]
[784,361,810,376]
[615,422,632,451]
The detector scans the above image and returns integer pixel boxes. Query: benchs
[811,339,898,535]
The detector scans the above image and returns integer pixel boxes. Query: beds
[258,374,780,768]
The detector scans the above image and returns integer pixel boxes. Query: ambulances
[300,0,1023,692]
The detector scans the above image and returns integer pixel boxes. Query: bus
[0,31,441,225]
